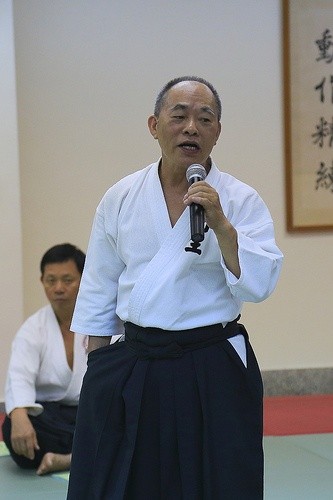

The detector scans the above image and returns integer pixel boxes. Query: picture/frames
[281,0,333,234]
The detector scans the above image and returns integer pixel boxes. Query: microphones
[187,164,206,242]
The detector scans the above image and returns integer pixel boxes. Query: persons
[67,77,285,500]
[1,242,122,475]
